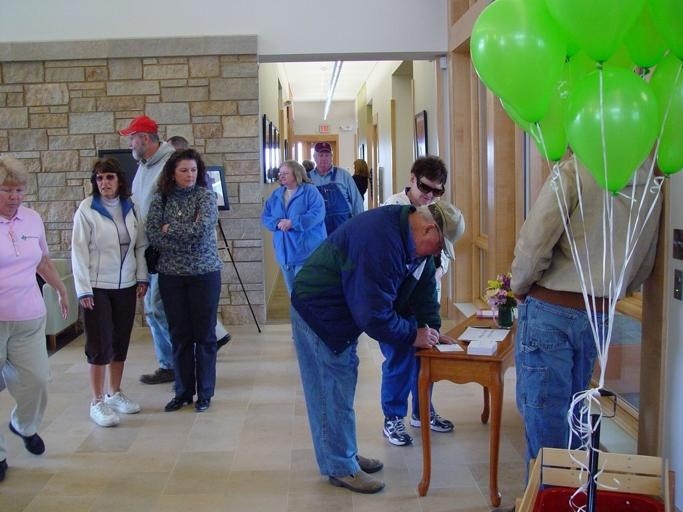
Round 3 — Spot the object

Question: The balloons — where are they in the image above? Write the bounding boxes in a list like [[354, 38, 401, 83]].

[[464, 1, 682, 196]]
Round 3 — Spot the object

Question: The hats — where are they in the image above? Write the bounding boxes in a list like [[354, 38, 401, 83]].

[[315, 142, 331, 153], [117, 116, 158, 136], [434, 200, 465, 262]]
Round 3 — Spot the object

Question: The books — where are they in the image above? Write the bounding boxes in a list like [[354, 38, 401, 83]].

[[475, 308, 519, 320]]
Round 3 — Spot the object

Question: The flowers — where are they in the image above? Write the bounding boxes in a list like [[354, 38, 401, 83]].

[[478, 270, 520, 310]]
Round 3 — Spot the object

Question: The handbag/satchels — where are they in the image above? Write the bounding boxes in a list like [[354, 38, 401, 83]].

[[145, 245, 160, 273]]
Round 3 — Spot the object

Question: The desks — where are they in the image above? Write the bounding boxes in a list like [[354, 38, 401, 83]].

[[412, 306, 519, 506]]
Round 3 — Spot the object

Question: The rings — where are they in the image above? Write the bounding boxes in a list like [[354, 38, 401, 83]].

[[84, 299, 88, 303]]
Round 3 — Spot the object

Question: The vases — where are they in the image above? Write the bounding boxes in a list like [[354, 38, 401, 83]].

[[492, 307, 514, 327]]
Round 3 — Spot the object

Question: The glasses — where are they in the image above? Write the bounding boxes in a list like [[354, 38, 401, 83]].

[[416, 177, 445, 197], [95, 175, 116, 181]]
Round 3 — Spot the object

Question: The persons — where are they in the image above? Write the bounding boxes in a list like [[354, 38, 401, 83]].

[[116, 115, 232, 413], [71, 154, 150, 428], [287, 199, 466, 495], [373, 155, 456, 449], [486, 150, 666, 512], [0, 155, 71, 486], [259, 138, 371, 341]]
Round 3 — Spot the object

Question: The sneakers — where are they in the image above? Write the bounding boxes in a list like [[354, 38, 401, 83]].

[[411, 411, 455, 432], [104, 392, 140, 414], [90, 400, 119, 426], [383, 416, 413, 446]]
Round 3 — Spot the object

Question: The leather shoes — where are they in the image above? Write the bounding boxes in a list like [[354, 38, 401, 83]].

[[329, 471, 385, 493], [357, 455, 383, 473], [139, 367, 176, 383], [215, 335, 231, 352], [164, 394, 211, 412], [8, 421, 45, 455], [0, 458, 8, 483]]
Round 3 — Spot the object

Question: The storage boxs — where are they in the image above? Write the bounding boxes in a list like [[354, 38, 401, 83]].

[[513, 445, 674, 512]]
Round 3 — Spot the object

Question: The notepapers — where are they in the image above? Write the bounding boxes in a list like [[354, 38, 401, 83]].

[[466, 340, 497, 355]]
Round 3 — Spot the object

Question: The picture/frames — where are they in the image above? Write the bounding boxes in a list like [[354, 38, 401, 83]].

[[204, 166, 230, 211], [263, 115, 290, 184], [413, 110, 428, 159]]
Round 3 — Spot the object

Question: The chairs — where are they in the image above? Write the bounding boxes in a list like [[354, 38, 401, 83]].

[[37, 257, 79, 352]]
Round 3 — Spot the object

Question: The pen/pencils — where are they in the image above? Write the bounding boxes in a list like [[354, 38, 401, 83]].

[[424, 323, 441, 346]]
